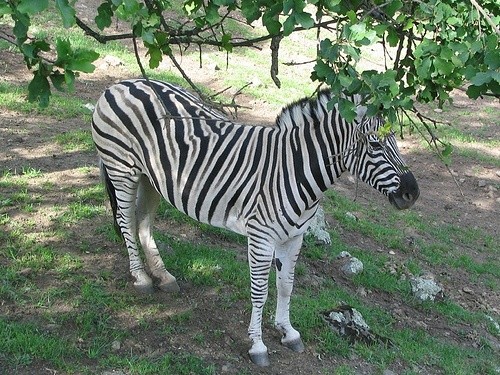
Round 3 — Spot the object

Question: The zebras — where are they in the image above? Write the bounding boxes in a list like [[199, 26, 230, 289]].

[[90, 77, 419, 367]]
